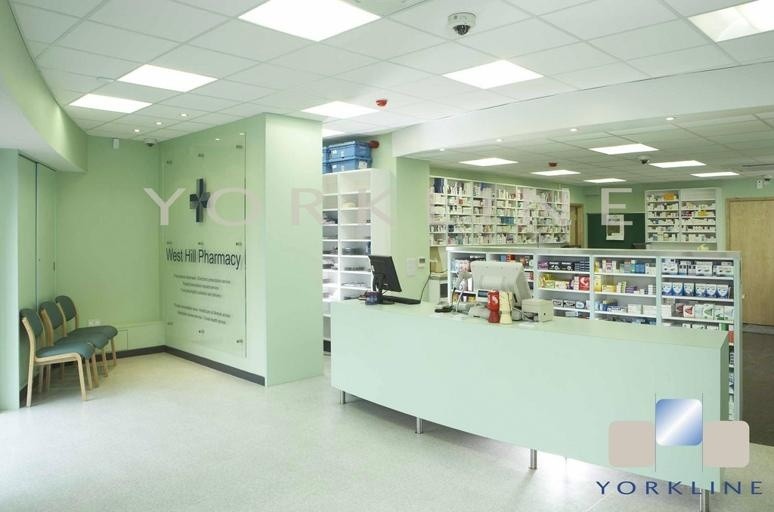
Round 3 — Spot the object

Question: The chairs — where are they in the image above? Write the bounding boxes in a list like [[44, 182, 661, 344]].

[[19, 296, 118, 407]]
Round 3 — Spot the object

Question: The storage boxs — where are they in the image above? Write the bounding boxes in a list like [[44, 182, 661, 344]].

[[321, 142, 373, 173]]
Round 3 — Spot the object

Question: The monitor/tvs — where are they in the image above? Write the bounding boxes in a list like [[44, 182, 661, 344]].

[[368, 254, 403, 304], [469, 261, 533, 307]]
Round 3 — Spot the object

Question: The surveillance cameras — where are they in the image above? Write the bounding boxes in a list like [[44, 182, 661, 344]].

[[450, 14, 474, 35], [639, 155, 650, 164]]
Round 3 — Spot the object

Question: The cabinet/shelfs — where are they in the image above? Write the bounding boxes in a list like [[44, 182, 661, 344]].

[[322, 167, 375, 342], [430, 177, 743, 421]]
